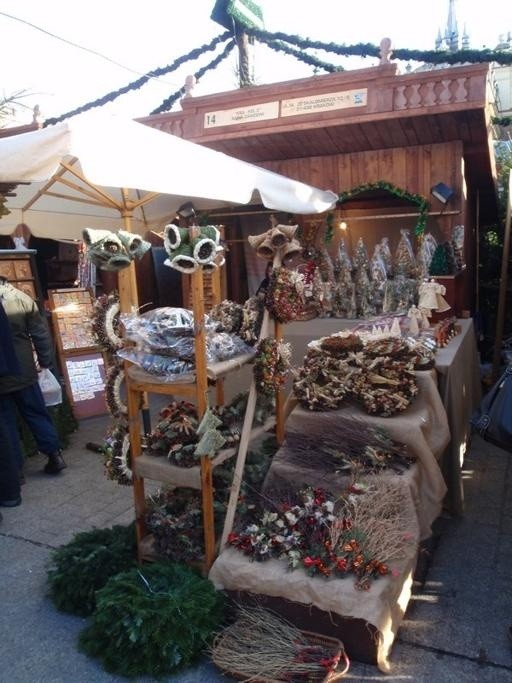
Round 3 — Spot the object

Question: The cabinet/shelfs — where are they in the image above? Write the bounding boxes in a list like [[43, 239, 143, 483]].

[[116, 226, 287, 580]]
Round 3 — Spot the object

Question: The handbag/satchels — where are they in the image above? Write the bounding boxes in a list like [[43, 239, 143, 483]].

[[469, 363, 512, 453]]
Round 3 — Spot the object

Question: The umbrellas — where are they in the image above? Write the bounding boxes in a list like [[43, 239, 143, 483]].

[[2, 103, 340, 450]]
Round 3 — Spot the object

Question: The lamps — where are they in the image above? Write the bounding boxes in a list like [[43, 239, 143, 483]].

[[430, 181, 455, 214]]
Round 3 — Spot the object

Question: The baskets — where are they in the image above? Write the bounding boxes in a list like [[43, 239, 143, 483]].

[[212, 624, 351, 682]]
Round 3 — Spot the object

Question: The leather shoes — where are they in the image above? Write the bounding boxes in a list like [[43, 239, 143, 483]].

[[0, 495, 22, 506], [44, 454, 67, 475]]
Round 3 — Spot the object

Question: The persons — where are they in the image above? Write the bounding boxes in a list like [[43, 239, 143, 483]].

[[0, 279, 69, 485], [0, 302, 23, 507]]
